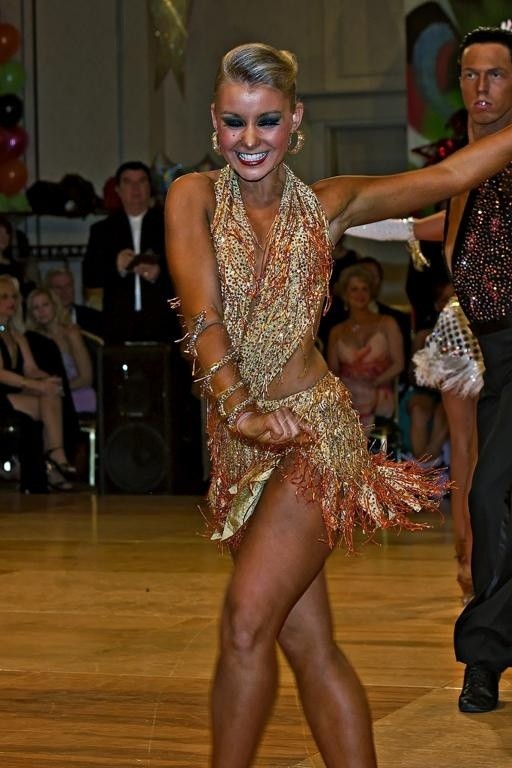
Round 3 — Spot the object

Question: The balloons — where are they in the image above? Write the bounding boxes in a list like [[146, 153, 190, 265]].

[[1, 18, 38, 213]]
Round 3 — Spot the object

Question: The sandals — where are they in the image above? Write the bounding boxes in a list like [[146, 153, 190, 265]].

[[49, 447, 77, 484], [45, 460, 74, 492]]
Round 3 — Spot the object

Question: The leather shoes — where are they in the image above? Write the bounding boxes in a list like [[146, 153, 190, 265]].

[[460, 661, 500, 714]]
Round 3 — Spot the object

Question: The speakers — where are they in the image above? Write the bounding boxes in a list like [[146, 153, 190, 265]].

[[95, 344, 200, 496]]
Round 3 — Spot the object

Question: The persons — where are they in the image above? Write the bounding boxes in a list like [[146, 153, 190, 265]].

[[2, 269, 104, 494], [313, 233, 450, 464], [84, 160, 205, 495], [165, 41, 512, 766], [440, 28, 512, 715], [338, 204, 492, 615]]
